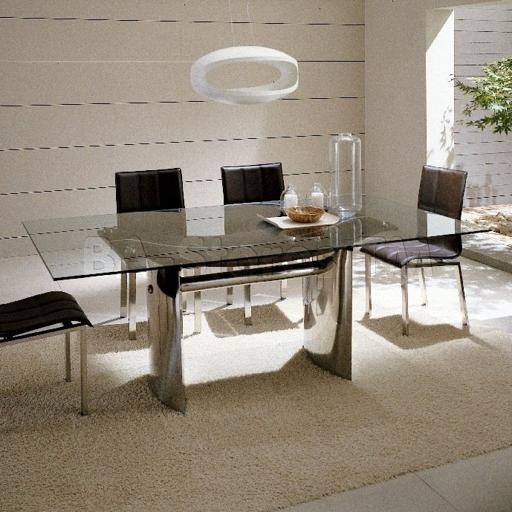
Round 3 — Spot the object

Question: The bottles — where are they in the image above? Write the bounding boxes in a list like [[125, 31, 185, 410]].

[[279, 181, 329, 216]]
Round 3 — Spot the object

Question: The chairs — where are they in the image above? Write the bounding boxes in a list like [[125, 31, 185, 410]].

[[106, 163, 289, 345], [359, 164, 470, 338]]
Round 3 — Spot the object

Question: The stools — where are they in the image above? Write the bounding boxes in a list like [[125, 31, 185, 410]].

[[0, 289, 93, 417]]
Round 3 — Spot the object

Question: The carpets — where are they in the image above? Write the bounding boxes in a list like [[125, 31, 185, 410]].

[[1, 285, 511, 511]]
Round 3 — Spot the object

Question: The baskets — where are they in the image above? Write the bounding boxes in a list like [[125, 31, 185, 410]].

[[286, 206, 323, 222]]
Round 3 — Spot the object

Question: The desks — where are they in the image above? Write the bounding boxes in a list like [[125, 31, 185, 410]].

[[19, 195, 485, 418]]
